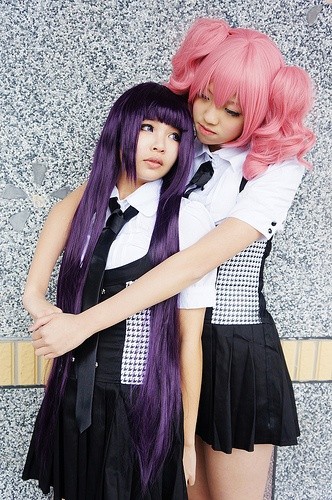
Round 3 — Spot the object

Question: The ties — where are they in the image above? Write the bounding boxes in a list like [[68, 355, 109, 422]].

[[180, 160, 217, 203], [72, 195, 144, 435]]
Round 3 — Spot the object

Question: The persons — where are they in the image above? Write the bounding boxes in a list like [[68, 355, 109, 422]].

[[20, 18, 316, 500], [19, 81, 216, 500]]
